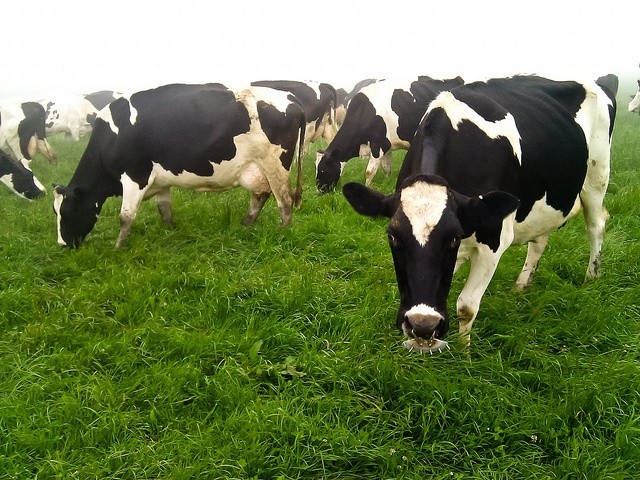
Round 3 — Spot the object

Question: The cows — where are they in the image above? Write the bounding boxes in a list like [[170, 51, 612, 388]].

[[38, 90, 123, 141], [251, 81, 337, 169], [335, 88, 349, 128], [315, 76, 465, 194], [0, 148, 47, 202], [52, 83, 305, 251], [0, 102, 58, 172], [345, 76, 434, 110], [342, 73, 619, 367]]
